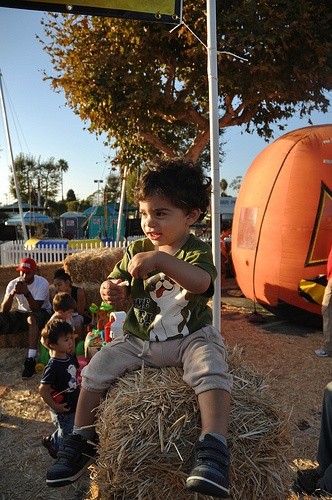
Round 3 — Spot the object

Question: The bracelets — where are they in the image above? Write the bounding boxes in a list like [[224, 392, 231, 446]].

[[24, 291, 32, 299]]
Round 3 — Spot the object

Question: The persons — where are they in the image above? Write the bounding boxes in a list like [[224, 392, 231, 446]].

[[293, 381, 332, 496], [220, 220, 232, 289], [45, 157, 234, 497], [314, 248, 332, 356], [0, 258, 88, 460]]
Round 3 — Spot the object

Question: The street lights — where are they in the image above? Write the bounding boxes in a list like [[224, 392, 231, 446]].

[[94, 180, 103, 206]]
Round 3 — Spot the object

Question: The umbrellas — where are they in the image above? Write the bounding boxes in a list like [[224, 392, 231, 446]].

[[0, 199, 139, 242]]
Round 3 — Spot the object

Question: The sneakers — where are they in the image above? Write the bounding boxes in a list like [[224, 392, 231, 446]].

[[21, 357, 36, 378], [314, 349, 329, 356], [43, 436, 59, 459], [46, 433, 98, 487], [185, 434, 230, 496]]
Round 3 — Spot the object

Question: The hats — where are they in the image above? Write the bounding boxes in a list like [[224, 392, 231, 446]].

[[16, 258, 36, 272]]
